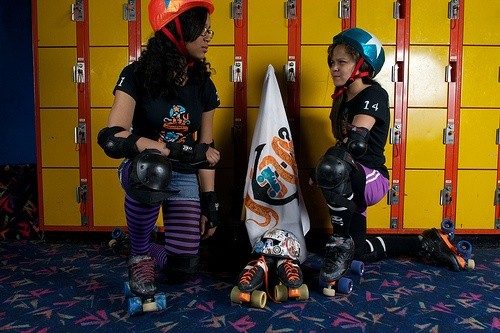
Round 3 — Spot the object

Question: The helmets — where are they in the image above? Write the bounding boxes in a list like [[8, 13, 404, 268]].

[[334, 28, 385, 78], [148, 0, 214, 32], [250, 229, 300, 258]]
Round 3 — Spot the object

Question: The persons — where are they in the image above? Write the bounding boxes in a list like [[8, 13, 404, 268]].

[[309, 28, 476, 296], [98, 0, 220, 313]]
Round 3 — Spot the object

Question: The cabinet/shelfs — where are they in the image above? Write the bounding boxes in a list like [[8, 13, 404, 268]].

[[34, 0, 500, 235]]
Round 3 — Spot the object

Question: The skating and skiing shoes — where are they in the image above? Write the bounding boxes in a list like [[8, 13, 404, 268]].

[[122, 247, 166, 315], [231, 257, 273, 308], [415, 219, 476, 272], [107, 223, 158, 251], [274, 258, 311, 302], [319, 234, 365, 296]]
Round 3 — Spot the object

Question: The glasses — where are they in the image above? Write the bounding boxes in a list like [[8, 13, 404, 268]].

[[201, 26, 214, 40]]
